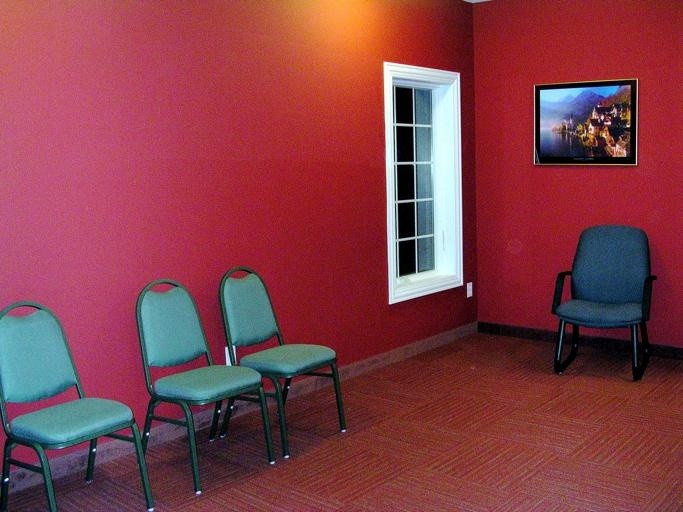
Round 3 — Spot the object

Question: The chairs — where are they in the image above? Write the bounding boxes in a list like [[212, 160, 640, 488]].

[[551, 223, 658, 382]]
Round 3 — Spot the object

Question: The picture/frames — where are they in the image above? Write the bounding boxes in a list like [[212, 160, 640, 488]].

[[533, 78, 640, 167]]
